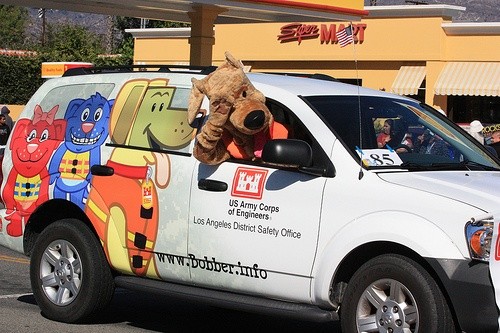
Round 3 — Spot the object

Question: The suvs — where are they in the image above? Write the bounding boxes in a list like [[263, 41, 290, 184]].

[[0, 63, 500, 333]]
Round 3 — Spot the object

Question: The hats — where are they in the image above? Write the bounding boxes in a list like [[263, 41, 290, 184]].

[[469, 120, 484, 133]]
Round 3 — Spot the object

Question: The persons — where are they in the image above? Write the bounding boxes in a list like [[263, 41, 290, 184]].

[[0, 106, 14, 154], [377, 119, 484, 160]]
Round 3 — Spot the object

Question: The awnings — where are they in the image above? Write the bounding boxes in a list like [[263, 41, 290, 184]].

[[434, 61, 500, 97], [391, 66, 426, 95]]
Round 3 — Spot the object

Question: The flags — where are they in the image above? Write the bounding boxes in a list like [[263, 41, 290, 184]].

[[336, 26, 353, 48]]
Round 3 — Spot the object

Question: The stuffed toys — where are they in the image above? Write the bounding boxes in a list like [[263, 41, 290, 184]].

[[187, 51, 288, 166]]
